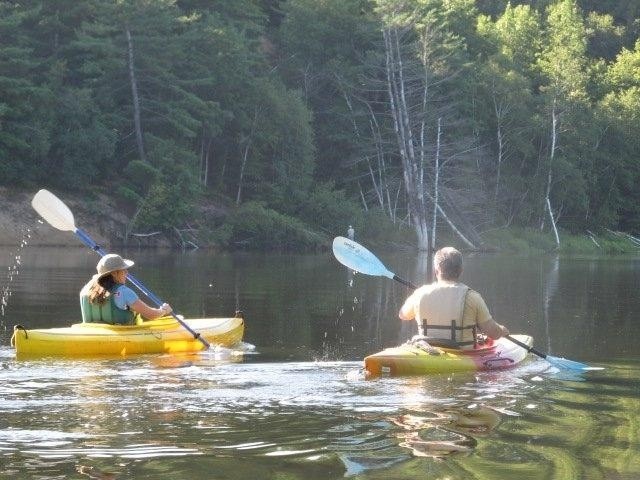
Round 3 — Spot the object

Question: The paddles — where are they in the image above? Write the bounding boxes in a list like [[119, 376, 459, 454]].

[[333, 236, 587, 370], [32, 188, 223, 353]]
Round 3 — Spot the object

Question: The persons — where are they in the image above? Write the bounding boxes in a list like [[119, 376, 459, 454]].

[[399, 247, 509, 351], [347, 226, 355, 241], [80, 254, 173, 326]]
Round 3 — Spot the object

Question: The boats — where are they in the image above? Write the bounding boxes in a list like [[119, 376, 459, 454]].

[[364, 334, 534, 375], [14, 318, 245, 362]]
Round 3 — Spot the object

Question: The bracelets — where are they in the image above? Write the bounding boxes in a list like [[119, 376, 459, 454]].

[[159, 305, 168, 315]]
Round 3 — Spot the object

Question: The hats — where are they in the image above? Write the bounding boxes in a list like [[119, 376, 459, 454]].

[[93, 254, 134, 282]]
